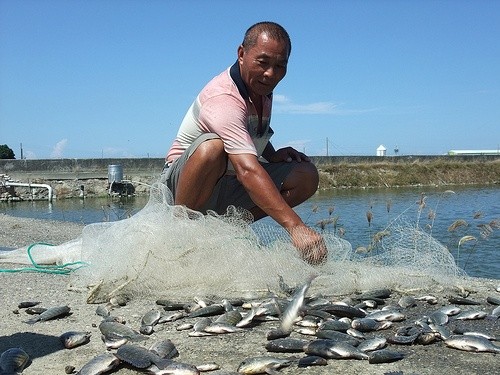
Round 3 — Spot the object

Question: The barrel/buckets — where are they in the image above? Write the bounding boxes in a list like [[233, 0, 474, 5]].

[[106, 164, 123, 195]]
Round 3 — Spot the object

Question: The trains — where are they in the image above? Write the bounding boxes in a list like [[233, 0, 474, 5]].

[[444, 149, 500, 154]]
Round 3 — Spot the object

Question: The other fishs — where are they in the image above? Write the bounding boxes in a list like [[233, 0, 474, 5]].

[[0, 273, 500, 375]]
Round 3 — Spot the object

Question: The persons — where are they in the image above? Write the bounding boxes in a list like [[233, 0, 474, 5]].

[[158, 22, 329, 268]]
